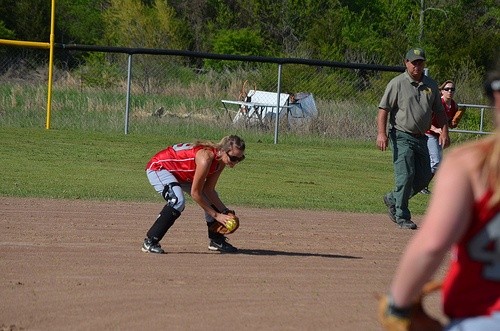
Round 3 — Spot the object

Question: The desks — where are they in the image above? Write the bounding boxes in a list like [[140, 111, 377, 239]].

[[221, 100, 295, 129]]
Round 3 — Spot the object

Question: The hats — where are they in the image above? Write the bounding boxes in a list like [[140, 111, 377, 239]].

[[405, 47, 425, 62]]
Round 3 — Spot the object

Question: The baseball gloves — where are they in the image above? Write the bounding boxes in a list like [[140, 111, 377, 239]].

[[209, 211, 240, 235]]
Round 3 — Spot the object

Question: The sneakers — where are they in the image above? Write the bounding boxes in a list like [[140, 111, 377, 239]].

[[384, 196, 396, 223], [141, 238, 166, 254], [207, 240, 238, 252], [420, 188, 431, 194], [398, 220, 417, 230]]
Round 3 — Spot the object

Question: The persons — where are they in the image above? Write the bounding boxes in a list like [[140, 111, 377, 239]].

[[376, 48, 441, 228], [141, 135, 246, 253], [379, 59, 500, 331], [421, 80, 460, 194]]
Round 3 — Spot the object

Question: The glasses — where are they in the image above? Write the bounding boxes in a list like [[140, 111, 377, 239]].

[[225, 151, 245, 162], [442, 87, 455, 91]]
